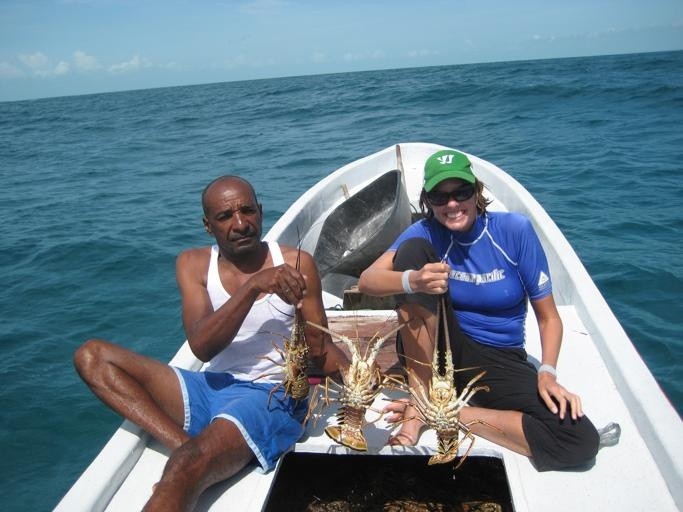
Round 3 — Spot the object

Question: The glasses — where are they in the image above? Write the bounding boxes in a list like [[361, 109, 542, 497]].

[[421, 184, 475, 208]]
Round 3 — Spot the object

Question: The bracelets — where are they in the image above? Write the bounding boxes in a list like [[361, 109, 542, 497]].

[[401, 269, 416, 295], [536, 364, 557, 378]]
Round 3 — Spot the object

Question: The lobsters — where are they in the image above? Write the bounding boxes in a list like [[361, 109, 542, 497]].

[[248, 224, 313, 423], [297, 277, 408, 454], [376, 231, 494, 481]]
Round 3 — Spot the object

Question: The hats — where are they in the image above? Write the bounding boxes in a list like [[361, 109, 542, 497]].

[[419, 148, 475, 194]]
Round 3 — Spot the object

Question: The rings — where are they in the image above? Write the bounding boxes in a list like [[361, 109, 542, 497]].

[[282, 288, 291, 295]]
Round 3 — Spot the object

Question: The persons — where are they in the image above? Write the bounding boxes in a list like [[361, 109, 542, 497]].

[[356, 148, 601, 474], [73, 175, 384, 512]]
